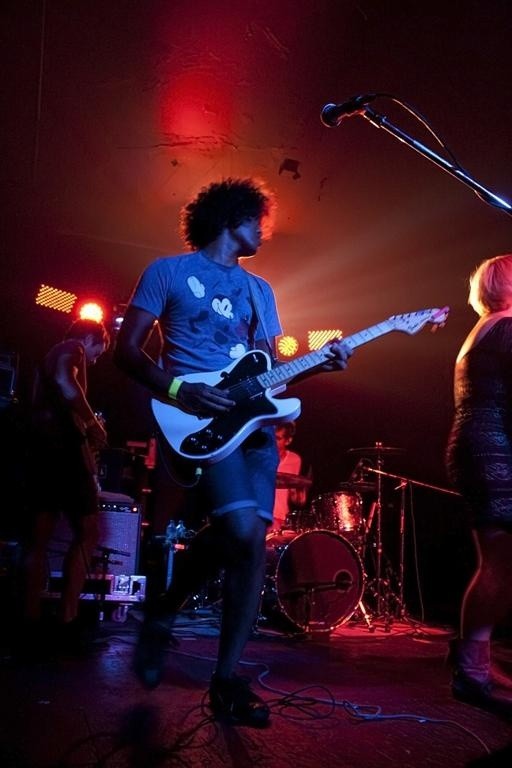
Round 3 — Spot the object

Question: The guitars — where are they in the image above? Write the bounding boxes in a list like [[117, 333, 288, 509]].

[[68, 407, 105, 477], [150, 306, 450, 463]]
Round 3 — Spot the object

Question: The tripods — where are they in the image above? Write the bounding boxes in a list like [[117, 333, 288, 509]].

[[363, 457, 412, 624]]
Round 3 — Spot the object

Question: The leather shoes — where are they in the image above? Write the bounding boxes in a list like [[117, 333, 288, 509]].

[[210, 672, 270, 724]]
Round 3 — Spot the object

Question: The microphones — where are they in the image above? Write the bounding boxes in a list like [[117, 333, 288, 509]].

[[348, 459, 364, 484], [320, 93, 374, 126]]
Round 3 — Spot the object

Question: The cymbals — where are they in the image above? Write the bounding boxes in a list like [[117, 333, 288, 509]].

[[341, 479, 378, 493], [272, 470, 312, 488], [342, 446, 406, 459]]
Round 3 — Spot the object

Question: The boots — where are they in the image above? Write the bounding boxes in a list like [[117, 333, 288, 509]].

[[449, 639, 511, 716]]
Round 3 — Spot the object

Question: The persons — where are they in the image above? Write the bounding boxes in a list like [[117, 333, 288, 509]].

[[112, 173, 357, 725], [268, 421, 313, 533], [441, 253, 510, 708], [327, 461, 393, 598], [16, 320, 115, 653]]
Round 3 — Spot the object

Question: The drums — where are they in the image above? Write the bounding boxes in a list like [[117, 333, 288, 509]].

[[309, 490, 366, 541], [261, 529, 367, 637]]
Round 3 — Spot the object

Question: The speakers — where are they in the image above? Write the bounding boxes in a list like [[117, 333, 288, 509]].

[[46, 492, 140, 594]]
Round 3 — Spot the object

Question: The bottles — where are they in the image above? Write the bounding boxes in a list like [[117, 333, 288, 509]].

[[165, 517, 185, 542]]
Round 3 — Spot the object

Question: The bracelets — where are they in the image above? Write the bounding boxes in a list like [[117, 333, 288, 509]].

[[168, 377, 184, 402], [86, 418, 98, 426]]
